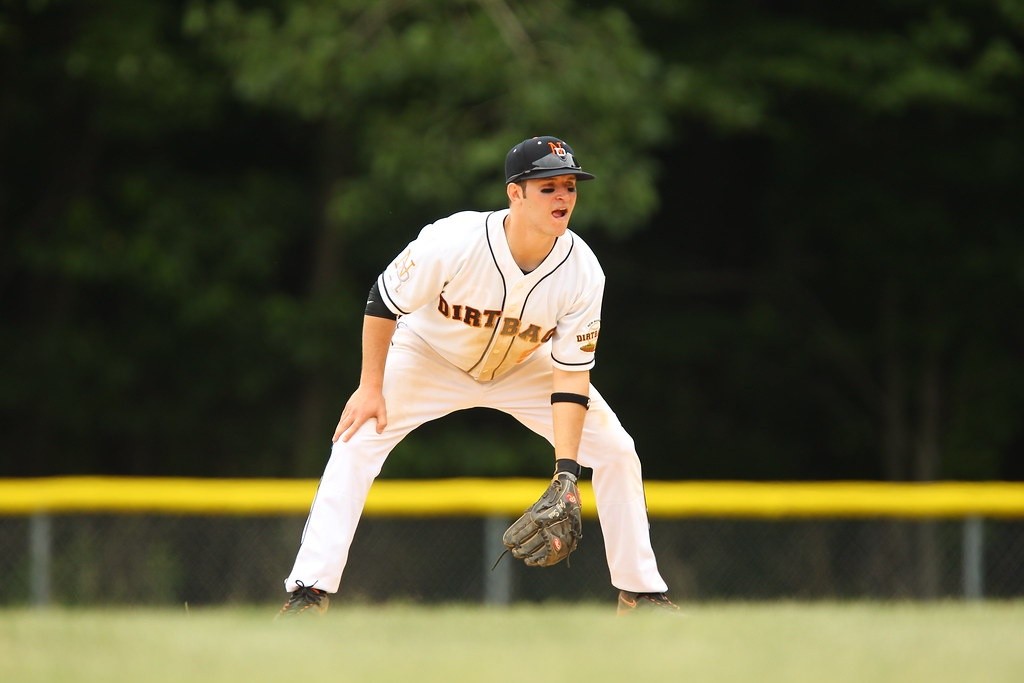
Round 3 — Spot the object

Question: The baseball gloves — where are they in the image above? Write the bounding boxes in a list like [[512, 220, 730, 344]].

[[488, 470, 585, 570]]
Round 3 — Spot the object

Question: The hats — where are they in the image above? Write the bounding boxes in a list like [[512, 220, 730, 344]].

[[505, 136, 595, 183]]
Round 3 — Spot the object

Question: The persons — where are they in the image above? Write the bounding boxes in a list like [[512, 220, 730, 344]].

[[270, 136, 680, 618]]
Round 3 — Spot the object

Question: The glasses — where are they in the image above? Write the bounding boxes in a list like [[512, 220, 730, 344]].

[[507, 151, 582, 182]]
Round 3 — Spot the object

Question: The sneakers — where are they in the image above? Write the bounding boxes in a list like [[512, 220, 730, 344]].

[[274, 579, 329, 625], [616, 591, 685, 619]]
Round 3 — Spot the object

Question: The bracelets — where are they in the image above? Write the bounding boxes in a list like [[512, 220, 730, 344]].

[[553, 458, 581, 476], [551, 392, 590, 408]]
